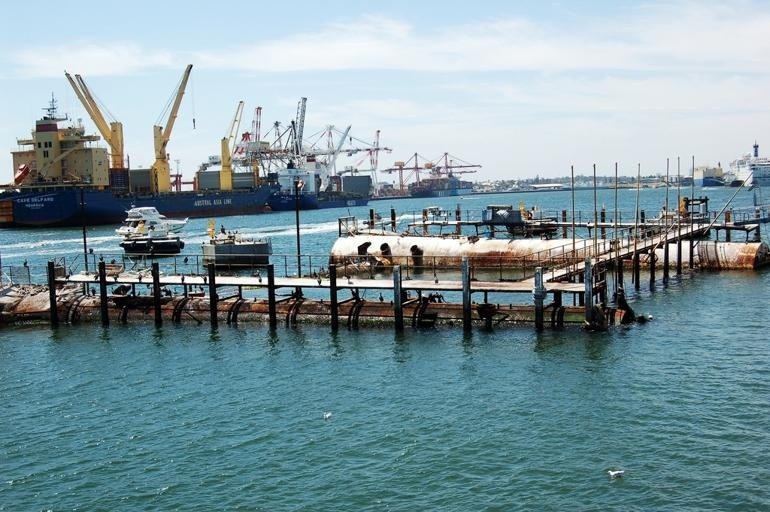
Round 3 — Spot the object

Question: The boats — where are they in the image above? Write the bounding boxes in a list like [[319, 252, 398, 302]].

[[506, 217, 559, 235], [116, 230, 185, 258], [197, 234, 273, 269], [116, 204, 189, 238]]
